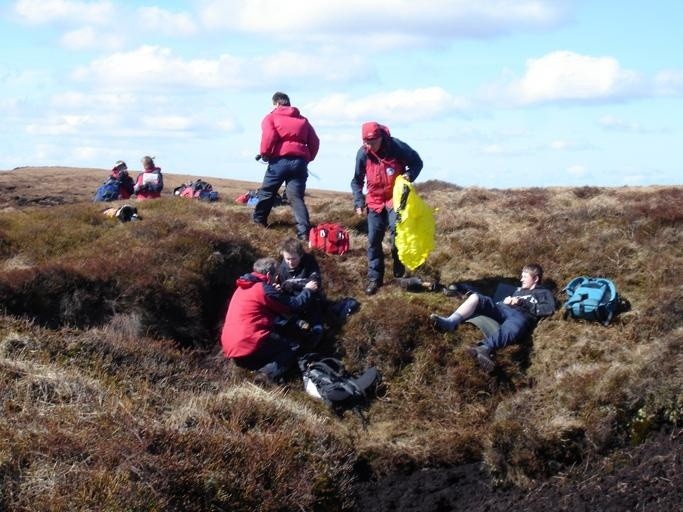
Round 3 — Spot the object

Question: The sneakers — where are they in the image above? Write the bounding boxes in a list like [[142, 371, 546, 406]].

[[401, 277, 420, 290], [365, 282, 382, 295], [296, 234, 308, 241], [250, 373, 276, 390], [394, 272, 407, 284], [469, 345, 495, 373]]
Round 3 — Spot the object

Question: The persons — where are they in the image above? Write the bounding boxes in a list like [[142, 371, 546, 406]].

[[251, 92, 319, 241], [352, 122, 423, 295], [133, 157, 162, 200], [430, 263, 555, 372], [275, 238, 322, 299], [221, 257, 318, 391], [104, 160, 133, 199]]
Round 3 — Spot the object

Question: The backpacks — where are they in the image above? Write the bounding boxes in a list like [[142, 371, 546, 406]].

[[560, 276, 618, 326], [298, 350, 379, 407], [95, 175, 123, 202], [173, 179, 217, 202], [308, 223, 349, 257], [235, 188, 292, 207]]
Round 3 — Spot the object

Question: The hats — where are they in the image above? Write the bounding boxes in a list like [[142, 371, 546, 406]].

[[363, 123, 380, 140], [112, 160, 127, 169], [139, 157, 155, 163]]
